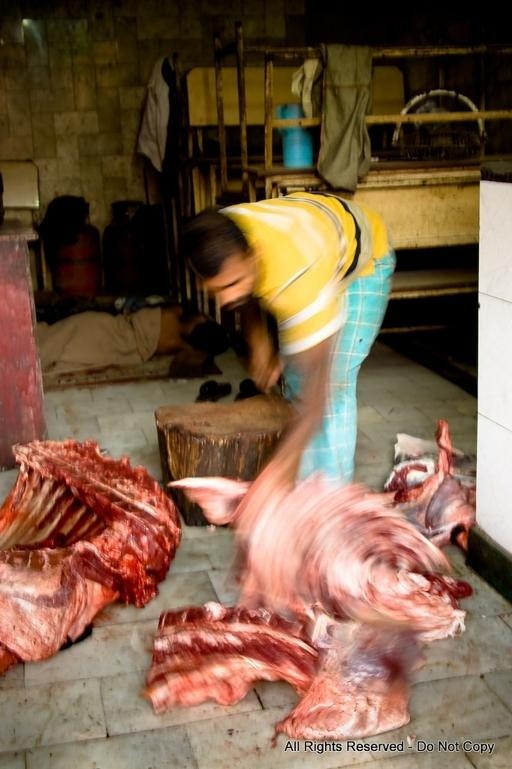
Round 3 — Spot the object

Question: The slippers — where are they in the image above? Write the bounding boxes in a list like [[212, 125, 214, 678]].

[[194, 380, 231, 404], [236, 378, 261, 398]]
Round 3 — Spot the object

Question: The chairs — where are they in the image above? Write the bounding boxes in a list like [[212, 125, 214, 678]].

[[1, 158, 45, 294]]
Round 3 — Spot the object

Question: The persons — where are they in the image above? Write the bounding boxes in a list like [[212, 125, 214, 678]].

[[179, 190, 399, 606]]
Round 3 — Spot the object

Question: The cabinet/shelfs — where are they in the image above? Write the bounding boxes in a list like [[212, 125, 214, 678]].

[[162, 28, 512, 338]]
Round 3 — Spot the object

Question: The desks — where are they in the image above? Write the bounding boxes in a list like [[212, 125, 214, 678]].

[[0, 218, 48, 471]]
